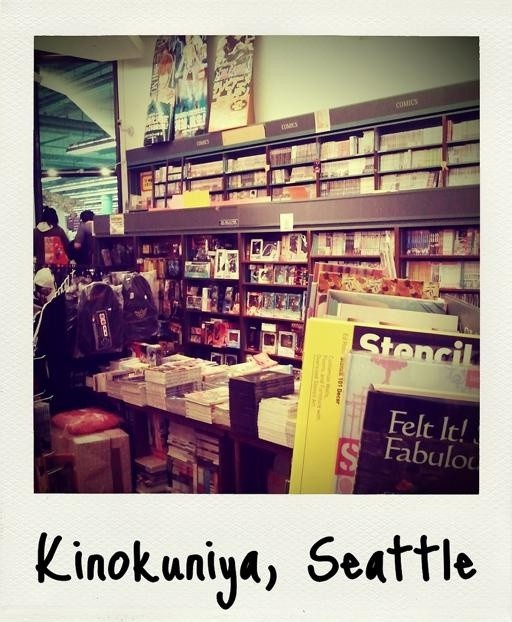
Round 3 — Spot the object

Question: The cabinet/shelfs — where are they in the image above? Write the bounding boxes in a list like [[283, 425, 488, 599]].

[[130, 405, 293, 494]]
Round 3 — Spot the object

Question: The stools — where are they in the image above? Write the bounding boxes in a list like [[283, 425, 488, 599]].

[[49, 427, 130, 493]]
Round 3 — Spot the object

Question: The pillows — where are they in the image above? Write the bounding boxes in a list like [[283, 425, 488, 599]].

[[52, 406, 123, 436]]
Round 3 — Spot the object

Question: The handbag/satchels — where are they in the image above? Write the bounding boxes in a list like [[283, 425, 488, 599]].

[[76, 281, 125, 358], [121, 274, 162, 343], [44, 236, 69, 266]]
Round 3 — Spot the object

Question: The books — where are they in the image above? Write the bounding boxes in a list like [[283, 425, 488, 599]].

[[142, 37, 255, 148], [80, 114, 479, 493]]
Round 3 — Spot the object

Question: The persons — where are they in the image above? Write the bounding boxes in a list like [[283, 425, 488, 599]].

[[68, 210, 96, 260], [34, 207, 76, 274]]
